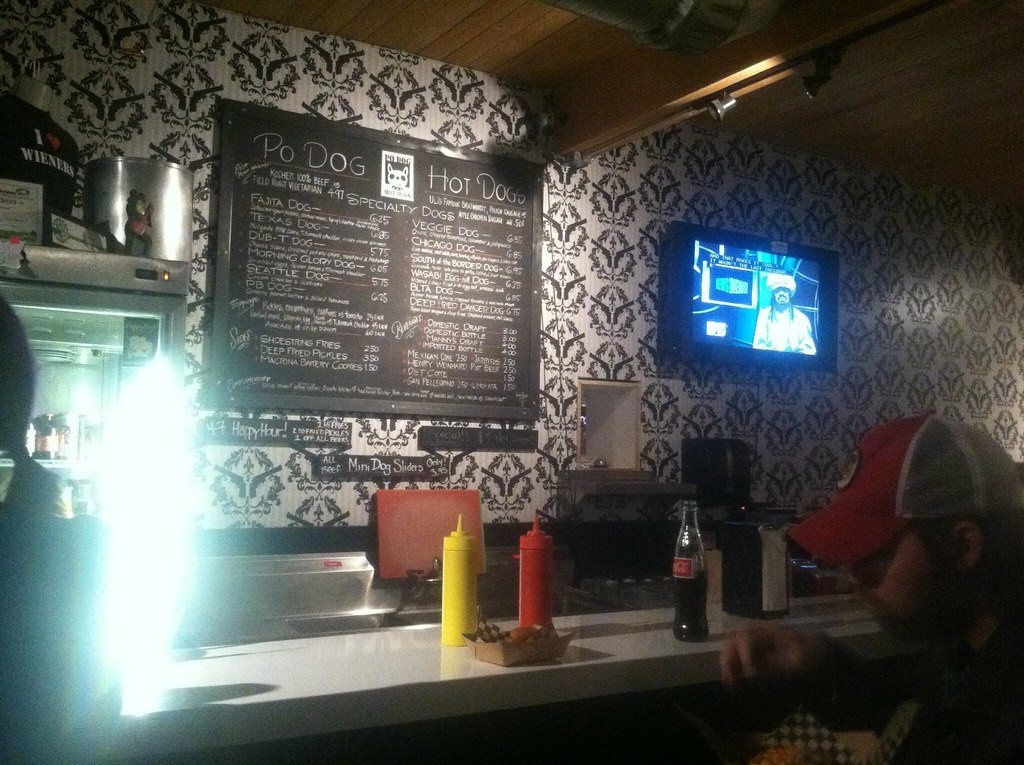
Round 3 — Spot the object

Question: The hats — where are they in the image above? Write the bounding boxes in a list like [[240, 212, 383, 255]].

[[787, 412, 1024, 566], [768, 274, 795, 300]]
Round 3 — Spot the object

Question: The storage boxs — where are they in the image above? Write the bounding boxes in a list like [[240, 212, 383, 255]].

[[461, 622, 574, 667]]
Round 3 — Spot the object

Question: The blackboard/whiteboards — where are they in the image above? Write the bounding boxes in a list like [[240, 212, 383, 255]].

[[316, 453, 450, 481], [200, 418, 353, 447], [204, 98, 544, 421], [418, 425, 539, 453]]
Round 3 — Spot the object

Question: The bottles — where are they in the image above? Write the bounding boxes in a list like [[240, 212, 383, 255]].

[[519, 513, 553, 629], [40, 412, 56, 459], [671, 501, 709, 641], [442, 514, 478, 648]]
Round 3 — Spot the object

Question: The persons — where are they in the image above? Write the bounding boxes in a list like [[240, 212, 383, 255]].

[[124, 189, 154, 257], [720, 414, 1024, 765], [753, 273, 817, 355], [0, 297, 121, 765]]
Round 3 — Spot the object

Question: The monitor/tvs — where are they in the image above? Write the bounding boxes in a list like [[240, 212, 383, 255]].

[[658, 222, 839, 374]]
